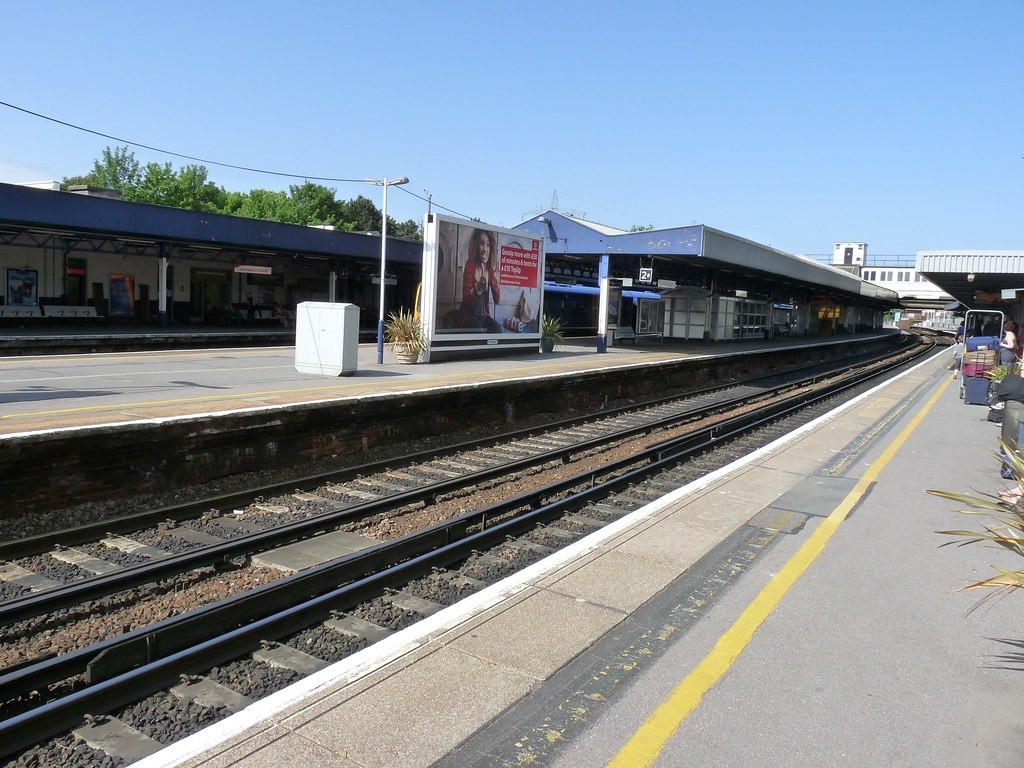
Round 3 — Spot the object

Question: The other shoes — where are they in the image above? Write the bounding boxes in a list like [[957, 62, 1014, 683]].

[[953, 375, 957, 379]]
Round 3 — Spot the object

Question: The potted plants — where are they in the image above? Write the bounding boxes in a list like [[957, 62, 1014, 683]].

[[380, 306, 431, 365], [539, 312, 567, 353]]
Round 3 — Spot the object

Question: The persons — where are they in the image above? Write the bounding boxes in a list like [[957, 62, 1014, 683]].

[[952, 315, 1024, 379], [459, 228, 502, 334]]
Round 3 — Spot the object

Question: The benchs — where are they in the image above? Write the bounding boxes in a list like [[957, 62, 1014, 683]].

[[614, 327, 637, 346]]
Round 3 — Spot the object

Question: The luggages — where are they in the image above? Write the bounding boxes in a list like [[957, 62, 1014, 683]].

[[963, 334, 1000, 386], [963, 353, 990, 405]]
